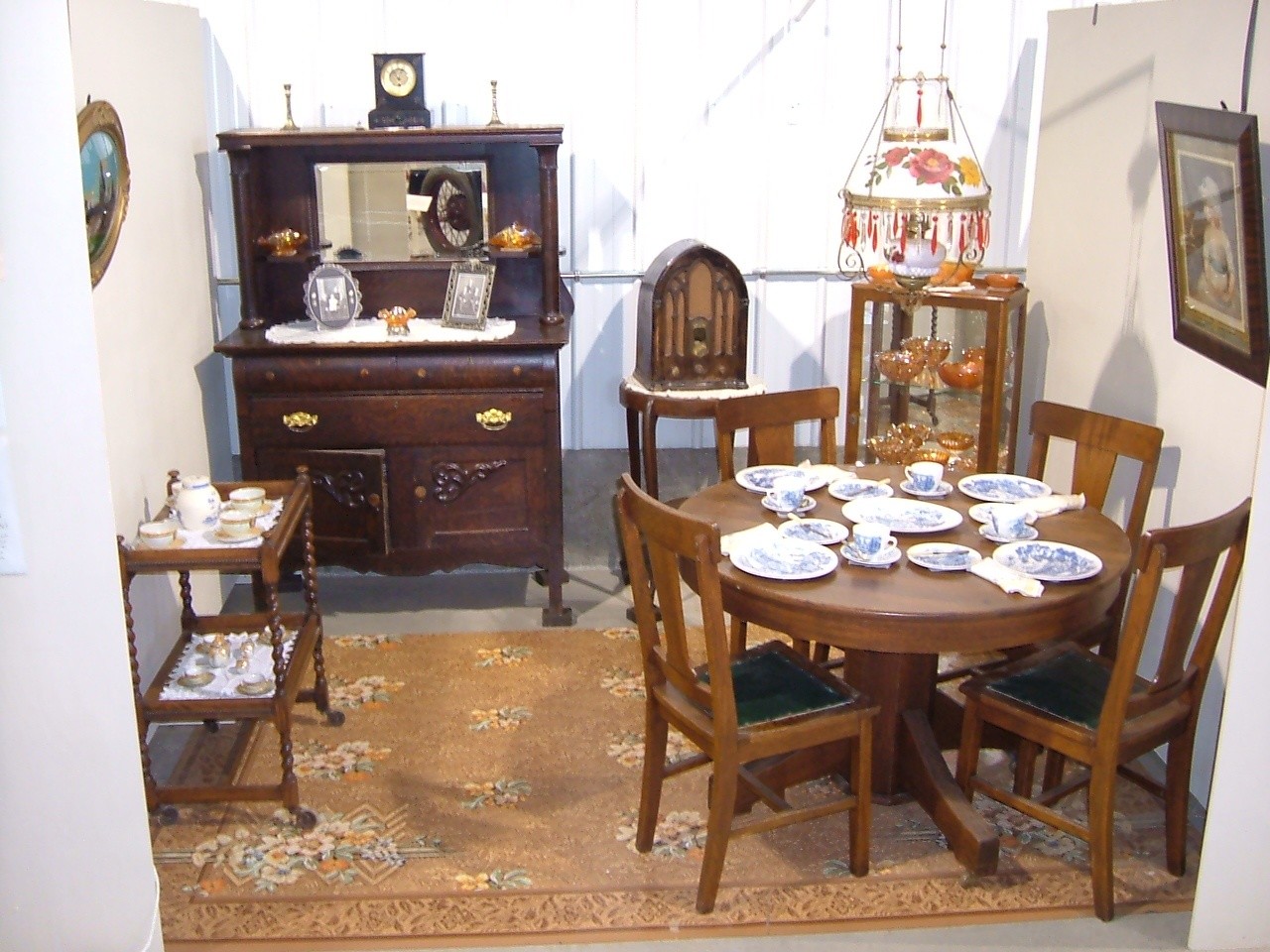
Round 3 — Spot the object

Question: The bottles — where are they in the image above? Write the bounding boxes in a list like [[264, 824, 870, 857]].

[[176, 475, 222, 534]]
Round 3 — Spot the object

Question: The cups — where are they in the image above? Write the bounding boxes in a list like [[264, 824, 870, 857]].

[[219, 510, 258, 536], [175, 623, 294, 695], [766, 476, 806, 507], [904, 461, 944, 492], [140, 520, 177, 550], [852, 522, 898, 560], [229, 487, 266, 511]]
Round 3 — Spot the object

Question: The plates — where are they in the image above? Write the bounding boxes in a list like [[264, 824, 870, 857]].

[[957, 474, 1053, 504], [979, 524, 1039, 544], [840, 542, 901, 569], [899, 479, 954, 498], [136, 502, 272, 550], [259, 630, 291, 645], [968, 502, 1039, 526], [213, 530, 259, 543], [196, 639, 231, 652], [734, 464, 827, 493], [827, 478, 894, 501], [761, 494, 816, 518], [177, 672, 214, 688], [841, 498, 964, 534], [992, 540, 1103, 582], [729, 539, 839, 580], [778, 519, 850, 545], [238, 679, 272, 695], [906, 543, 982, 570]]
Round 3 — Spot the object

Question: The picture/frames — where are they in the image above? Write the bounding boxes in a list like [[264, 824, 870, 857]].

[[77, 100, 131, 290], [440, 256, 497, 332], [303, 262, 363, 334], [1155, 101, 1270, 389]]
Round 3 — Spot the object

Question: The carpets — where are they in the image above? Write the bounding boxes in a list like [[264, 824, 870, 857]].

[[139, 620, 1204, 952]]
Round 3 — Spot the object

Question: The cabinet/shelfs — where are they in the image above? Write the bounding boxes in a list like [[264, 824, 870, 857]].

[[843, 273, 1028, 475], [213, 127, 575, 627]]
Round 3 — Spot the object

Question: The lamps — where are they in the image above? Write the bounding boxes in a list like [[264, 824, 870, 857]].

[[834, 0, 993, 317]]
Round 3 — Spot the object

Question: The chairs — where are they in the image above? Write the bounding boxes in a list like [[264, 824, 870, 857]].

[[613, 471, 886, 916], [955, 496, 1258, 922], [933, 398, 1164, 802], [714, 383, 849, 682]]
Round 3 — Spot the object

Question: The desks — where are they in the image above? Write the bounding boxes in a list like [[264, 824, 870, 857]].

[[676, 464, 1135, 879], [618, 377, 764, 627]]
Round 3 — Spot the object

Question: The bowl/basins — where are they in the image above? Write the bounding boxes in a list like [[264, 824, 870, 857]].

[[865, 262, 1019, 466]]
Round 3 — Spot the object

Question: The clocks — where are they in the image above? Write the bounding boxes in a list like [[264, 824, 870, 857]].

[[367, 53, 430, 130]]
